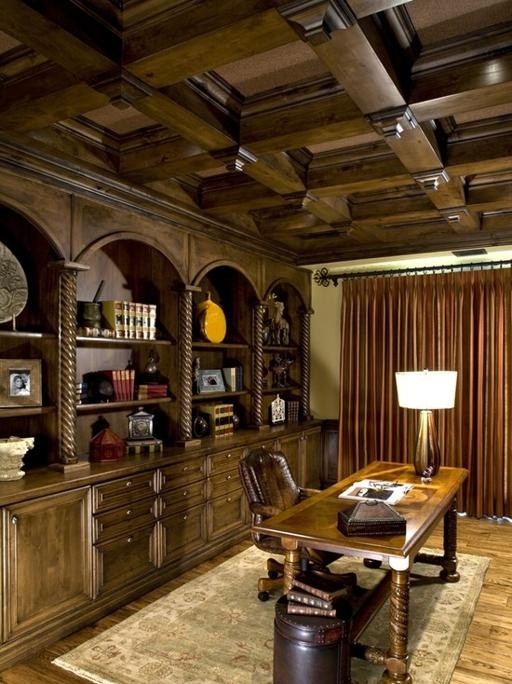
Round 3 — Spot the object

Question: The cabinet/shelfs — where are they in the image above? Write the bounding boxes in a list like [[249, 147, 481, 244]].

[[0, 134, 326, 672]]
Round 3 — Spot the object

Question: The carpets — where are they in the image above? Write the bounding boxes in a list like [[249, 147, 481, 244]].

[[50, 545, 493, 684]]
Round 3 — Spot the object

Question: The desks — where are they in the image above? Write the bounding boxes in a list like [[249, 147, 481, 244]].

[[253, 459, 473, 683]]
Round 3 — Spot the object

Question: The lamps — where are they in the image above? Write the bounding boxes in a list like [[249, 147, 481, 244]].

[[393, 366, 459, 474]]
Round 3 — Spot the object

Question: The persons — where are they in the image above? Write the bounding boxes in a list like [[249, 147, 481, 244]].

[[11, 373, 30, 395], [132, 418, 149, 437]]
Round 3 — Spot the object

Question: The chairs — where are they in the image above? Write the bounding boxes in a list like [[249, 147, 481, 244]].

[[238, 447, 357, 602]]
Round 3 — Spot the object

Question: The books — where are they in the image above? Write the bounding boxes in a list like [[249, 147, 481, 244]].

[[285, 570, 349, 618]]
